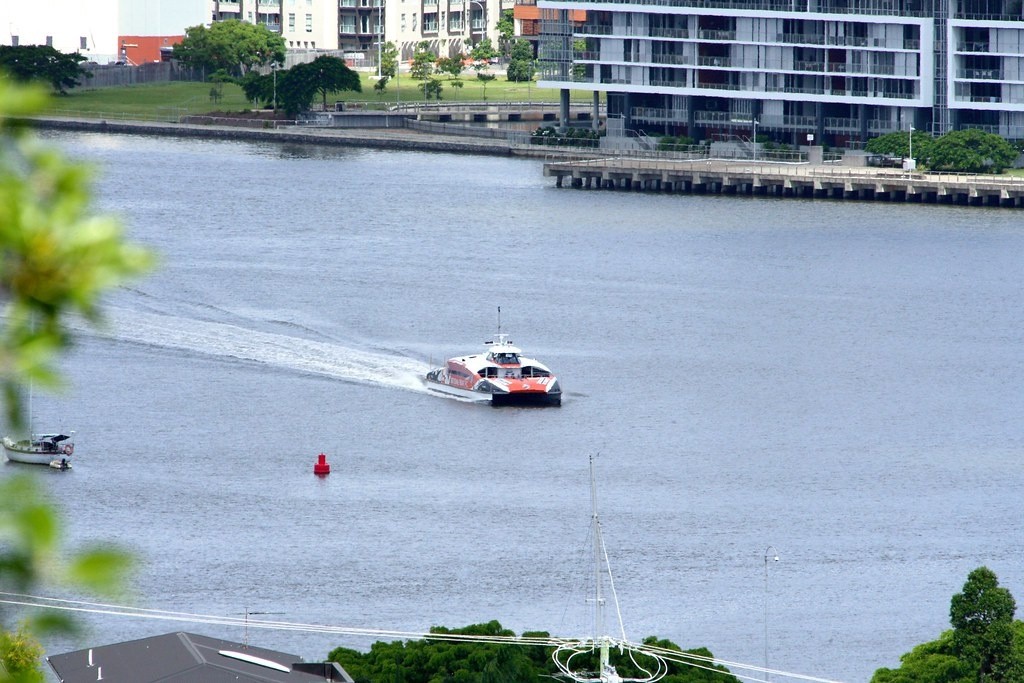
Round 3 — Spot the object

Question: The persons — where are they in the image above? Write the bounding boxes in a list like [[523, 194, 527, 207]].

[[492, 352, 520, 364]]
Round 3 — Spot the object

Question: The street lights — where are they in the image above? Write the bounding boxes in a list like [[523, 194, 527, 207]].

[[908, 123, 916, 158], [753, 117, 760, 161], [471, 0, 484, 43]]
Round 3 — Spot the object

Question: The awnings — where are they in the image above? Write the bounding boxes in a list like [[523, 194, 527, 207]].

[[32, 434, 71, 444]]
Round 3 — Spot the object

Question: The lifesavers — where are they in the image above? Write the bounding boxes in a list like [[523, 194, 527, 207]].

[[64, 445, 73, 455]]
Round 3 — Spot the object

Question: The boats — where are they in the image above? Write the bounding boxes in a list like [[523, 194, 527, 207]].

[[50, 459, 73, 471], [425, 305, 564, 408]]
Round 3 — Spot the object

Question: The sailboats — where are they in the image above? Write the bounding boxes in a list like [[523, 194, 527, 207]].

[[0, 300, 77, 465]]
